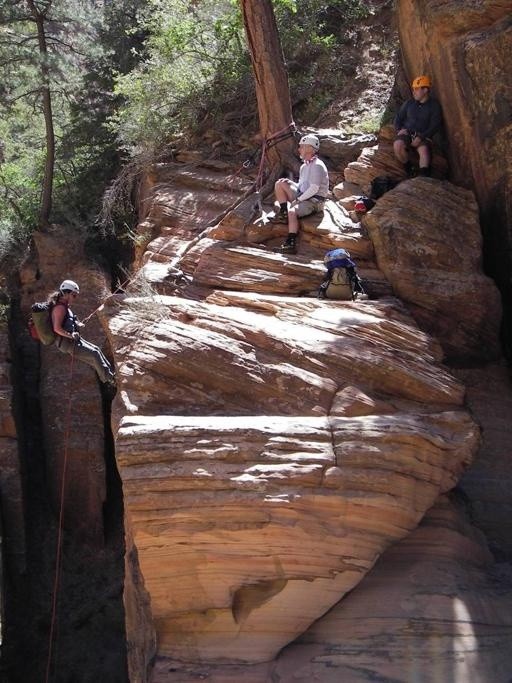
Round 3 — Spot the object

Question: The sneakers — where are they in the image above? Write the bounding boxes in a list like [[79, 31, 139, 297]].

[[267, 212, 288, 224], [272, 239, 297, 254], [406, 158, 430, 177]]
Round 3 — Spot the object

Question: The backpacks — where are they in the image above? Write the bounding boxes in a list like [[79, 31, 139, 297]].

[[318, 247, 369, 302], [30, 302, 69, 346]]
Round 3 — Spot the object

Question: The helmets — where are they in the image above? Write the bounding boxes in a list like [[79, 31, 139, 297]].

[[411, 75, 432, 88], [60, 279, 80, 294], [299, 133, 320, 149]]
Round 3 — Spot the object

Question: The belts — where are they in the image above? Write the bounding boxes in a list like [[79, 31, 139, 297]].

[[297, 188, 325, 201]]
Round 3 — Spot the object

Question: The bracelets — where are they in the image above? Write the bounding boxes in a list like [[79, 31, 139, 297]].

[[296, 197, 299, 204]]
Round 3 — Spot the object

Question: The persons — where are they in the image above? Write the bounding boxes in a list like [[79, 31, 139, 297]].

[[393, 75, 443, 179], [48, 279, 117, 388], [270, 134, 330, 255]]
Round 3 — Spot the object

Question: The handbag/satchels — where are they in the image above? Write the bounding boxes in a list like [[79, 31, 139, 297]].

[[367, 175, 396, 196]]
